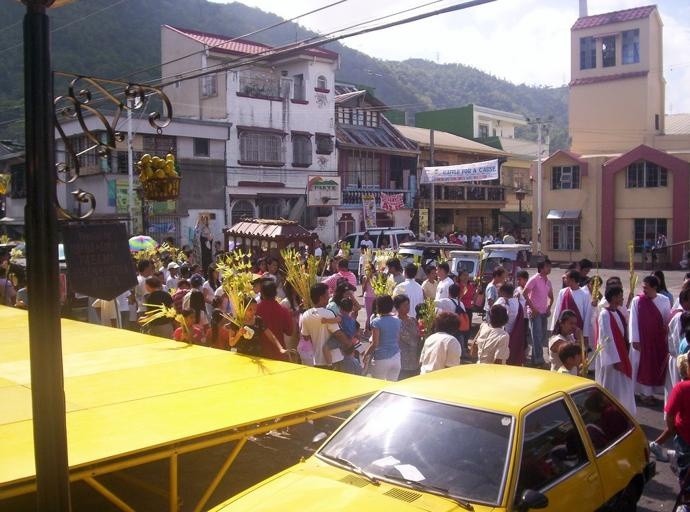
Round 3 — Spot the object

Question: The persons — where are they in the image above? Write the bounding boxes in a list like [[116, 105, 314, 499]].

[[92, 238, 293, 361], [643, 237, 654, 260], [467, 255, 602, 376], [298, 232, 473, 382], [593, 270, 690, 511], [1, 264, 29, 310], [424, 229, 528, 250], [651, 231, 668, 261]]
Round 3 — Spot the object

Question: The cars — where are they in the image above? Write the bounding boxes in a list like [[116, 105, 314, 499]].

[[204, 362, 657, 512]]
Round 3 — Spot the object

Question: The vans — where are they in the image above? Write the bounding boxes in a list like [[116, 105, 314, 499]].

[[327, 227, 418, 284]]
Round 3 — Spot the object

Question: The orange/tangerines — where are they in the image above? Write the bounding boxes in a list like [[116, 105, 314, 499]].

[[138, 154, 178, 197]]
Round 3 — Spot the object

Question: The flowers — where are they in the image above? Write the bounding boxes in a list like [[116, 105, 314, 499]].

[[417, 296, 439, 339], [624, 237, 640, 309], [471, 270, 486, 293], [214, 247, 254, 337], [577, 330, 610, 380], [361, 245, 449, 296], [131, 240, 190, 339], [588, 238, 604, 307], [279, 241, 350, 309]]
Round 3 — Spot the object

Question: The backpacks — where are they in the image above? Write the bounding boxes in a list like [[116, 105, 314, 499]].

[[451, 298, 469, 331]]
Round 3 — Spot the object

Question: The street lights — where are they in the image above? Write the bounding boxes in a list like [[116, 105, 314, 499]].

[[125, 87, 142, 239], [537, 127, 551, 258], [515, 187, 527, 242]]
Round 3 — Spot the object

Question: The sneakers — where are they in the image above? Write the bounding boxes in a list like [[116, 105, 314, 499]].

[[649, 441, 668, 461]]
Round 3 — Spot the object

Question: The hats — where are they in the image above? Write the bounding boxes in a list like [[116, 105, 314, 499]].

[[191, 275, 202, 286], [168, 262, 180, 270]]
